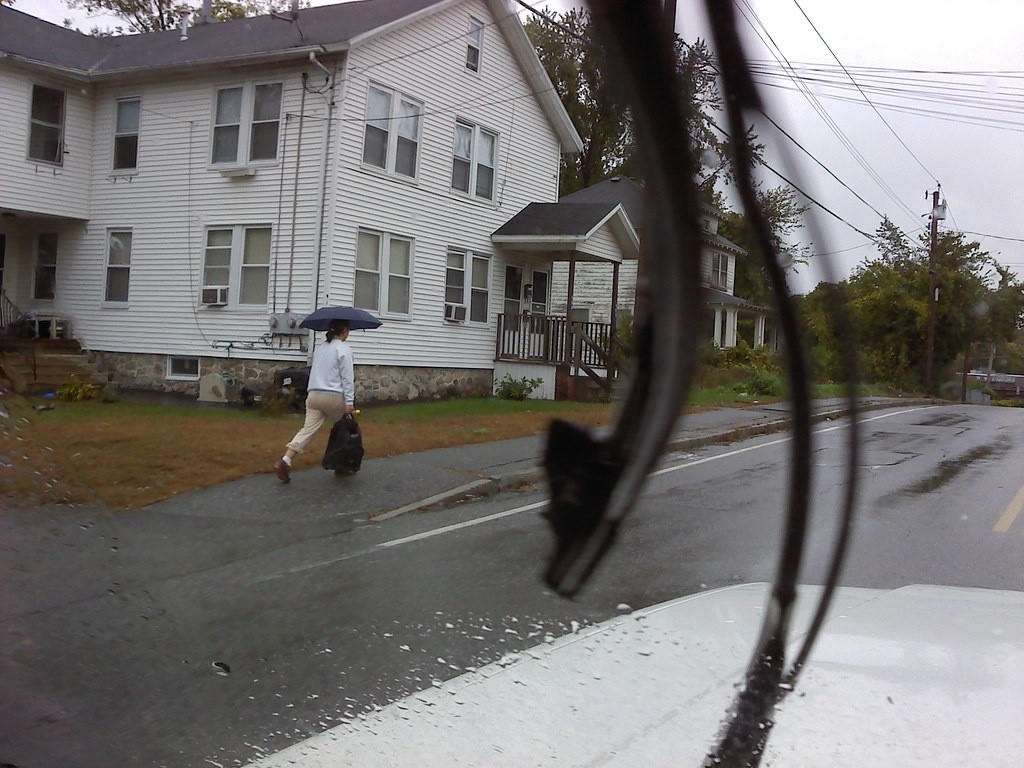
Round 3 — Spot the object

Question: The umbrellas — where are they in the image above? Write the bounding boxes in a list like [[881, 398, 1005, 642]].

[[298, 306, 382, 331]]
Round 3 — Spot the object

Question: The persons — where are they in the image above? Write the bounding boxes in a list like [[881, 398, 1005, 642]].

[[274, 320, 354, 482]]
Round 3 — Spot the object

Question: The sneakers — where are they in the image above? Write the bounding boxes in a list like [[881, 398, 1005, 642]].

[[274, 459, 291, 484]]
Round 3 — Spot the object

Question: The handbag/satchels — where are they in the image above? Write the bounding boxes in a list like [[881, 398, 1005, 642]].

[[322, 412, 364, 472]]
